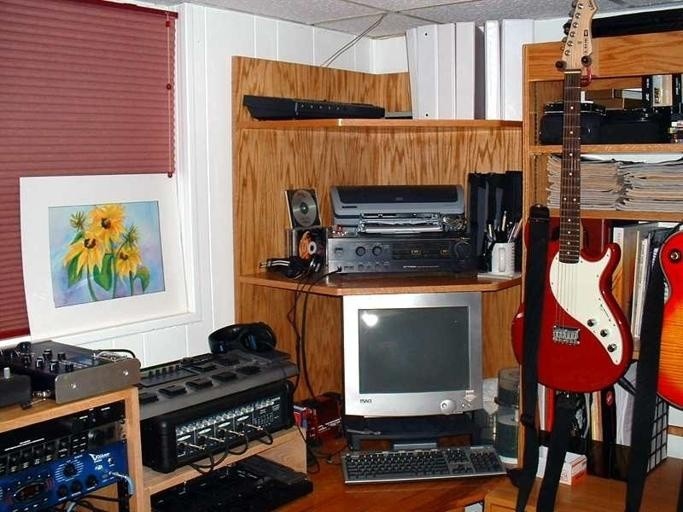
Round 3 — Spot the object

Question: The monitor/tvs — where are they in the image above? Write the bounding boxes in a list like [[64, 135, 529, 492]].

[[342, 291, 484, 440]]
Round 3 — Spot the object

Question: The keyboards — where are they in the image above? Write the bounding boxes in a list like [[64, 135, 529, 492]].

[[243, 95, 385, 119], [340, 445, 507, 484]]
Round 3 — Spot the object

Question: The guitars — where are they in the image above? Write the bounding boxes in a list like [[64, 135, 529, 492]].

[[656, 230, 683, 409], [511, 0, 634, 391]]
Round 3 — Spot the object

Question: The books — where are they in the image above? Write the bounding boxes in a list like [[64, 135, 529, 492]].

[[545, 155, 683, 213], [589, 222, 680, 446]]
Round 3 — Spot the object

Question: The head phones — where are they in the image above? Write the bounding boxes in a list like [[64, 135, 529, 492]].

[[259, 254, 321, 280], [208, 321, 277, 355]]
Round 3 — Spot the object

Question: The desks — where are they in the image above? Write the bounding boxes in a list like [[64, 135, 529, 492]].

[[486, 456, 683, 512], [1, 384, 508, 512]]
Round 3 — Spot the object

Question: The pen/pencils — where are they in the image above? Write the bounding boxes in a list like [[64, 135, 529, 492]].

[[488, 211, 523, 243]]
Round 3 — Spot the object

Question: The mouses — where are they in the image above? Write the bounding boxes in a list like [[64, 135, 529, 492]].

[[508, 468, 522, 488]]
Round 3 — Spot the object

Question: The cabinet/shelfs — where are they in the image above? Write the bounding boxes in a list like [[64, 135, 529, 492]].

[[230, 55, 524, 427], [517, 30, 683, 469]]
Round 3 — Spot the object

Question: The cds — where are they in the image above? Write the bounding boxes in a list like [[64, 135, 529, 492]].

[[291, 190, 317, 227]]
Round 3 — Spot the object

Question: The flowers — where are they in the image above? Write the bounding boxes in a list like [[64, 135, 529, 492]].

[[62, 204, 151, 301]]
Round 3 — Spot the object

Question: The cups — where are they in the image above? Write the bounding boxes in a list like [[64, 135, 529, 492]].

[[490, 242, 516, 276]]
